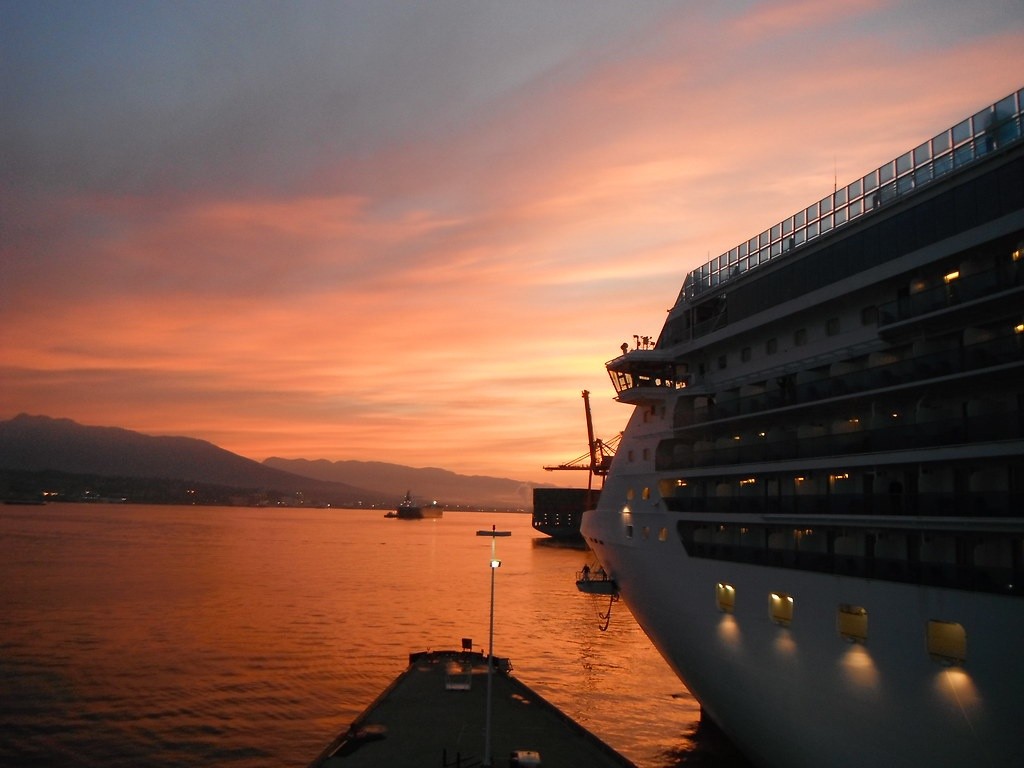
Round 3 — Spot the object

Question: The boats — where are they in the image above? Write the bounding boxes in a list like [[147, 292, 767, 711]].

[[384, 489, 444, 519], [576, 561, 619, 594], [311, 536, 635, 767], [476, 529, 511, 537], [532, 390, 626, 539]]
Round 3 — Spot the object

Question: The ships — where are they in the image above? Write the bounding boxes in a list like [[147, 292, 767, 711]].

[[581, 89, 1024, 768]]
